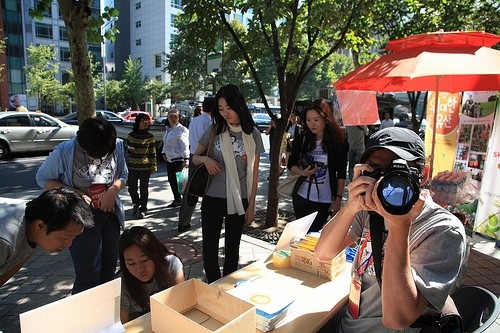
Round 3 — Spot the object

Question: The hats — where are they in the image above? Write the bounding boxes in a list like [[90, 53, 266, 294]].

[[167, 109, 179, 118], [360, 128, 427, 165]]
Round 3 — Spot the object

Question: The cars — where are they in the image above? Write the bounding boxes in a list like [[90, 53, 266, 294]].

[[119, 110, 154, 125], [155, 115, 169, 124], [0, 106, 82, 159], [57, 109, 130, 125], [253, 113, 273, 132]]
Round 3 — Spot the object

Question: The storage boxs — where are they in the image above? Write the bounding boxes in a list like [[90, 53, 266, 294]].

[[289, 246, 346, 281], [149, 278, 255, 333]]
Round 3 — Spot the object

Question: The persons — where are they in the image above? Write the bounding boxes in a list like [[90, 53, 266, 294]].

[[178, 97, 214, 231], [315, 127, 466, 333], [163, 109, 190, 207], [380, 112, 407, 129], [465, 95, 481, 118], [119, 226, 185, 323], [266, 110, 300, 179], [460, 123, 491, 181], [0, 188, 95, 288], [193, 84, 265, 284], [125, 113, 157, 218], [347, 125, 373, 179], [287, 99, 347, 233], [36, 117, 128, 295]]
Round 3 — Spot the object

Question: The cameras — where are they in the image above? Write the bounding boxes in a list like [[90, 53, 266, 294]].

[[363, 159, 424, 216], [299, 153, 325, 170]]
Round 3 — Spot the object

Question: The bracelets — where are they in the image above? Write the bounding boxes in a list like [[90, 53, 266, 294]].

[[336, 195, 342, 198]]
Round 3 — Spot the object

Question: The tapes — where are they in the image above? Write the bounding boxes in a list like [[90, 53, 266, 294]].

[[272, 250, 290, 269]]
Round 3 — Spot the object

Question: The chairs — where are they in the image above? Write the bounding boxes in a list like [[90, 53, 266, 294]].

[[444, 284, 499, 333]]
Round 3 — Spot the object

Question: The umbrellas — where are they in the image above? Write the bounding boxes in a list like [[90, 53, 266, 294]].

[[333, 29, 500, 182]]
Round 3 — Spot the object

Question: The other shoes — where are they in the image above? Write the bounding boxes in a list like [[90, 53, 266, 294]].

[[167, 201, 181, 207]]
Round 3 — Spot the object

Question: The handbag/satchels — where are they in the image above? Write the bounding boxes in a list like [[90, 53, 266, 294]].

[[277, 174, 297, 199], [185, 164, 211, 198], [176, 168, 188, 194]]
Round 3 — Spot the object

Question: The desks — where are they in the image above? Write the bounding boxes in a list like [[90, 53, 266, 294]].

[[122, 252, 356, 333]]
